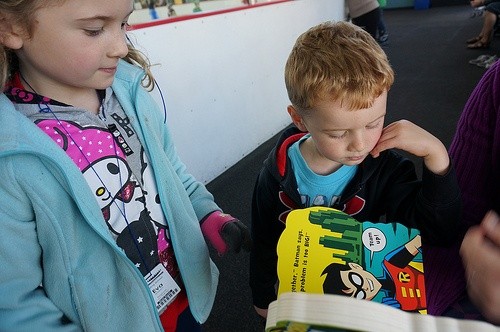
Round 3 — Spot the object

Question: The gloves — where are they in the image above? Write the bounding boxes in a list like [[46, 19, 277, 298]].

[[199, 209, 249, 260]]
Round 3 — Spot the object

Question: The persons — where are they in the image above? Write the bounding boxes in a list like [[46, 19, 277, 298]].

[[0, 0, 249, 332], [426, 0, 500, 331], [249, 20, 459, 332]]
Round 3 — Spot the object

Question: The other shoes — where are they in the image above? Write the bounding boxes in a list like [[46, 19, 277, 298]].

[[466, 35, 488, 49], [469, 55, 498, 68], [379, 33, 390, 44]]
